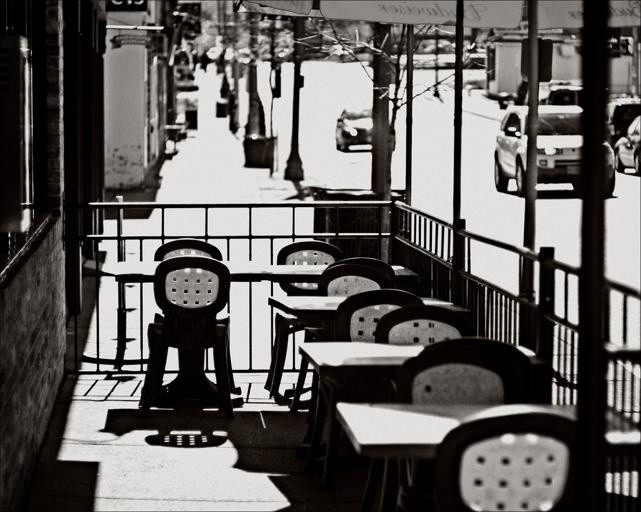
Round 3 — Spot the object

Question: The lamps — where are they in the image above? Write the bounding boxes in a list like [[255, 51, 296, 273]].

[[303, 0, 328, 37]]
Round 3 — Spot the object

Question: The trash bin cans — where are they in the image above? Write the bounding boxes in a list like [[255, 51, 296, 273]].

[[309, 186, 405, 260]]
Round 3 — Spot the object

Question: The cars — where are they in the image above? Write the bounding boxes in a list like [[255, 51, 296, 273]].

[[344, 34, 488, 70], [335, 108, 396, 155], [492, 77, 640, 199]]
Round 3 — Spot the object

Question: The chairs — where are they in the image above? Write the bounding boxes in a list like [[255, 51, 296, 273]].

[[428, 412, 609, 511], [265, 241, 344, 400], [153, 237, 243, 394], [331, 287, 426, 346], [139, 257, 236, 417], [376, 305, 476, 353], [280, 257, 396, 426], [403, 336, 541, 409]]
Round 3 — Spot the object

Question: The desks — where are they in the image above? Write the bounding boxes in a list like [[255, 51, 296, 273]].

[[98, 254, 418, 392], [265, 289, 458, 414], [332, 392, 639, 509], [292, 331, 542, 489]]
